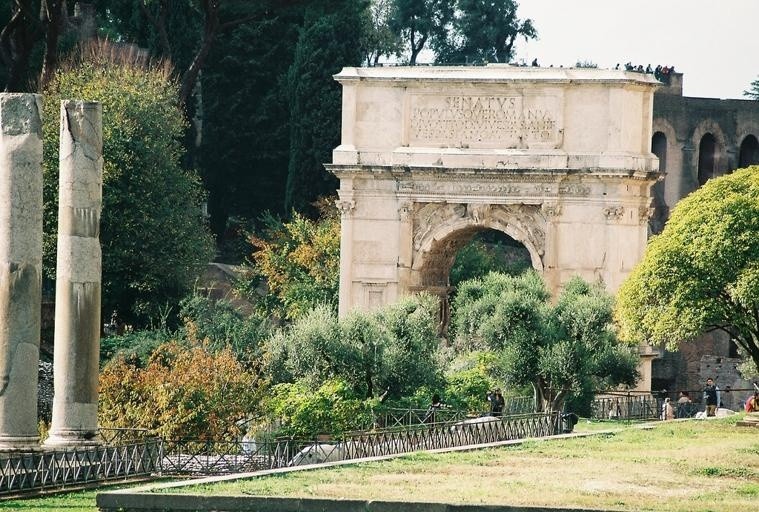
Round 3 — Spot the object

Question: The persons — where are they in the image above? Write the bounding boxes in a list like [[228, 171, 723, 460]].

[[745, 390, 759, 412], [704, 378, 720, 417], [487, 388, 505, 417], [662, 391, 691, 421], [426, 394, 453, 422]]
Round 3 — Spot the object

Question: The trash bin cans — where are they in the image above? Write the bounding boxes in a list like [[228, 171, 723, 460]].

[[562, 414, 573, 432]]
